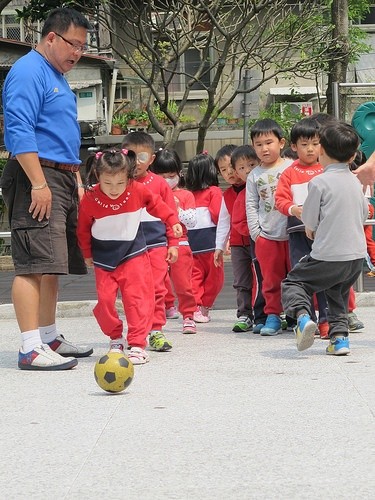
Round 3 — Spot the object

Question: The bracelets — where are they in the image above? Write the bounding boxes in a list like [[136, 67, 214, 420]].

[[77, 184, 85, 189], [31, 183, 48, 191]]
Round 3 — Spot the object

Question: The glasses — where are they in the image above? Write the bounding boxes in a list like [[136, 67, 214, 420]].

[[134, 152, 153, 163], [54, 31, 90, 55]]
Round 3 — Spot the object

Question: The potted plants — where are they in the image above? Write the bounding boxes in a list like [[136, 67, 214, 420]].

[[111, 103, 239, 135]]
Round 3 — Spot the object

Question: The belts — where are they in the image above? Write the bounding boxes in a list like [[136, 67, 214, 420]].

[[9, 153, 81, 174]]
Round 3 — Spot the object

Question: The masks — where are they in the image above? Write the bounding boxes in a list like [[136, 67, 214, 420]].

[[165, 174, 180, 189]]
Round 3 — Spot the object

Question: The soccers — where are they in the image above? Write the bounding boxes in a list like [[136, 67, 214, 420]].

[[94, 351, 135, 393]]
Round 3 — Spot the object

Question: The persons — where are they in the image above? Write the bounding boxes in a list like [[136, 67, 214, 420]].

[[350, 149, 375, 202], [304, 113, 365, 331], [279, 124, 367, 356], [245, 120, 320, 338], [347, 148, 375, 277], [163, 269, 180, 321], [212, 143, 255, 334], [273, 118, 331, 340], [77, 147, 180, 367], [183, 152, 226, 325], [123, 129, 179, 355], [0, 8, 94, 372], [148, 149, 196, 335], [230, 146, 300, 336]]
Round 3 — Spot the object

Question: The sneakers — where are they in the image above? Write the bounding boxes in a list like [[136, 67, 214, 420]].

[[149, 331, 172, 352], [108, 336, 126, 350], [183, 317, 196, 333], [192, 310, 209, 323], [233, 311, 365, 339], [124, 345, 150, 365], [325, 337, 350, 355], [198, 305, 211, 320], [19, 344, 78, 371], [165, 305, 179, 318], [47, 334, 93, 358], [292, 314, 317, 351]]
